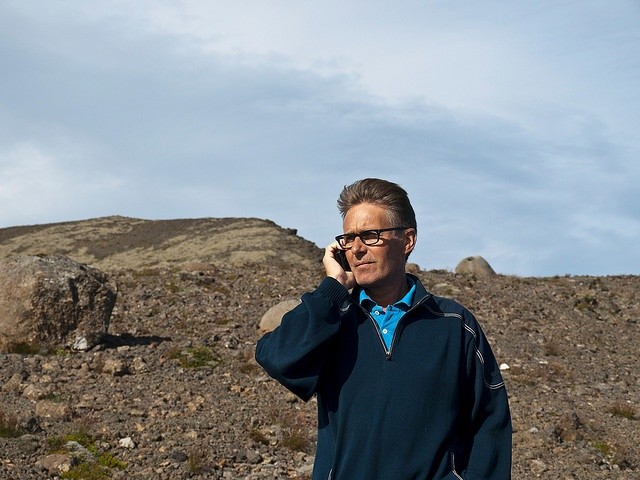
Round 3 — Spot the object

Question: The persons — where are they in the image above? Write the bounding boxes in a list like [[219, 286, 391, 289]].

[[255, 176, 512, 479]]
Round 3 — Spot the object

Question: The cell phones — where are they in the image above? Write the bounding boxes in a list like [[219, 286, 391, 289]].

[[333, 250, 351, 271]]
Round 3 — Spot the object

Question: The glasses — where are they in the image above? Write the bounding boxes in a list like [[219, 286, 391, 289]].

[[335, 226, 415, 249]]
